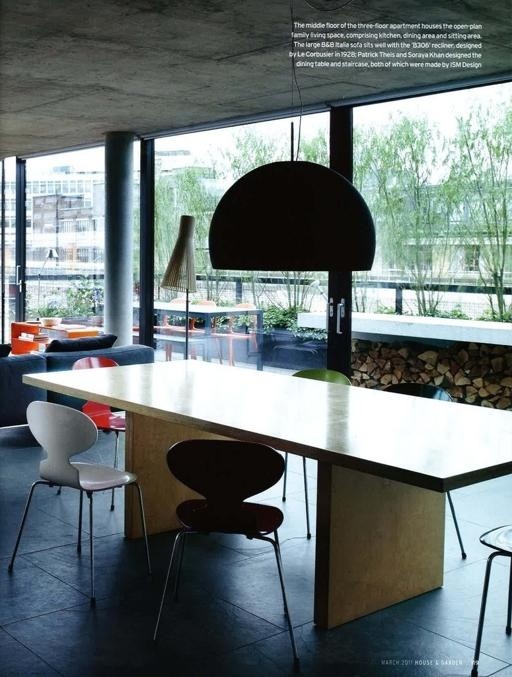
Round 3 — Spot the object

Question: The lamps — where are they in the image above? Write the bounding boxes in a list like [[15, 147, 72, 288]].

[[159, 214, 196, 361], [209, 0, 376, 270], [36, 248, 59, 324]]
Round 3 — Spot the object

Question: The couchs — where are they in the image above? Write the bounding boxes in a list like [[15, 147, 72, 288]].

[[0, 343, 156, 427]]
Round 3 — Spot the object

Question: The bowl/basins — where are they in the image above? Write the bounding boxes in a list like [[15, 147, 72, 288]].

[[40, 317, 63, 327]]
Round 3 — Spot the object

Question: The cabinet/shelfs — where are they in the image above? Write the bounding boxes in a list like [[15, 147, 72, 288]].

[[10, 321, 98, 355]]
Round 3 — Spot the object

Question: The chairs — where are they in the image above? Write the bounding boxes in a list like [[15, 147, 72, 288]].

[[133, 296, 264, 371]]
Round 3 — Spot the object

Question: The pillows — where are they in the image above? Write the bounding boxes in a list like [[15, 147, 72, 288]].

[[45, 333, 117, 352]]
